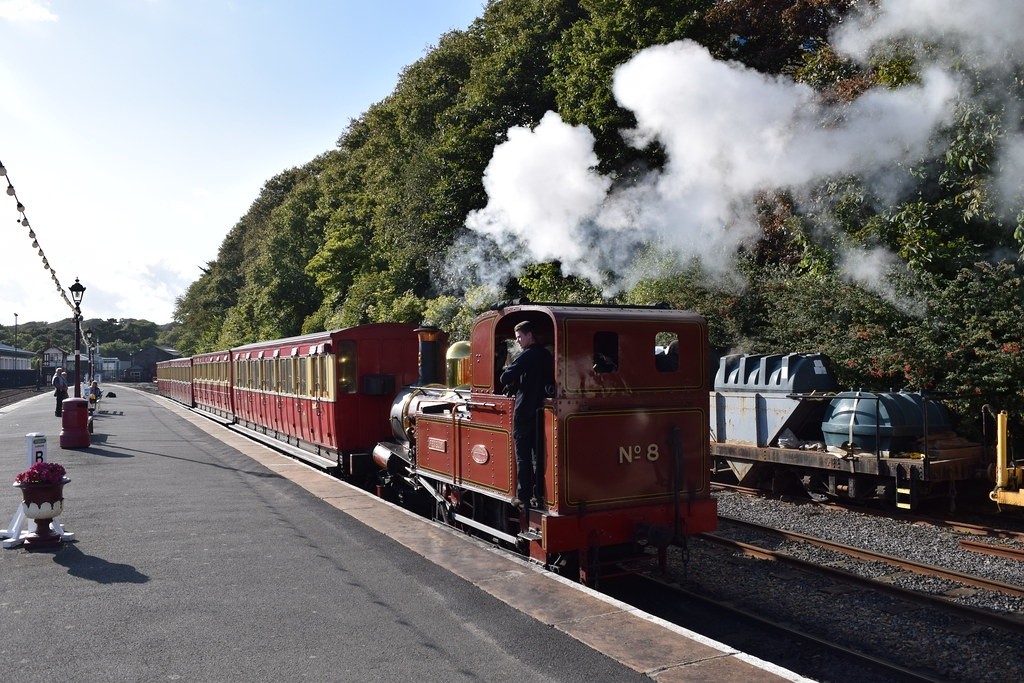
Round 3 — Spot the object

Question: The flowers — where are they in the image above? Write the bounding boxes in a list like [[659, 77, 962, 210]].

[[15, 462, 66, 485]]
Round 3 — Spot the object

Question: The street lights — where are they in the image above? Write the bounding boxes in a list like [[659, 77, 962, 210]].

[[85, 328, 92, 386], [67, 277, 86, 400], [13, 312, 18, 387], [90, 343, 96, 382]]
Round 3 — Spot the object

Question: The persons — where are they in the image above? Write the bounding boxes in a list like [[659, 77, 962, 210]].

[[52, 367, 70, 416], [89, 380, 102, 415], [500, 319, 555, 512]]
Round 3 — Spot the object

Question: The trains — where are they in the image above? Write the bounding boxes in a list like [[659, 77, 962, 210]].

[[154, 303, 720, 593], [706, 354, 988, 523]]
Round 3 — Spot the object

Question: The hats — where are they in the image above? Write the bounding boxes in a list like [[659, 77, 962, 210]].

[[61, 372, 67, 375]]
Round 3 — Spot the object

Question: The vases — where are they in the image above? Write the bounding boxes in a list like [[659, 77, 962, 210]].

[[13, 477, 72, 550]]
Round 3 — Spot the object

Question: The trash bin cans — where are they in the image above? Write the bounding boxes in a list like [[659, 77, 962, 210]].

[[59, 397, 91, 448]]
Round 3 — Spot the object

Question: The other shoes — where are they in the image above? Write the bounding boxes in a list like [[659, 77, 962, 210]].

[[530, 497, 543, 509], [511, 496, 530, 510]]
